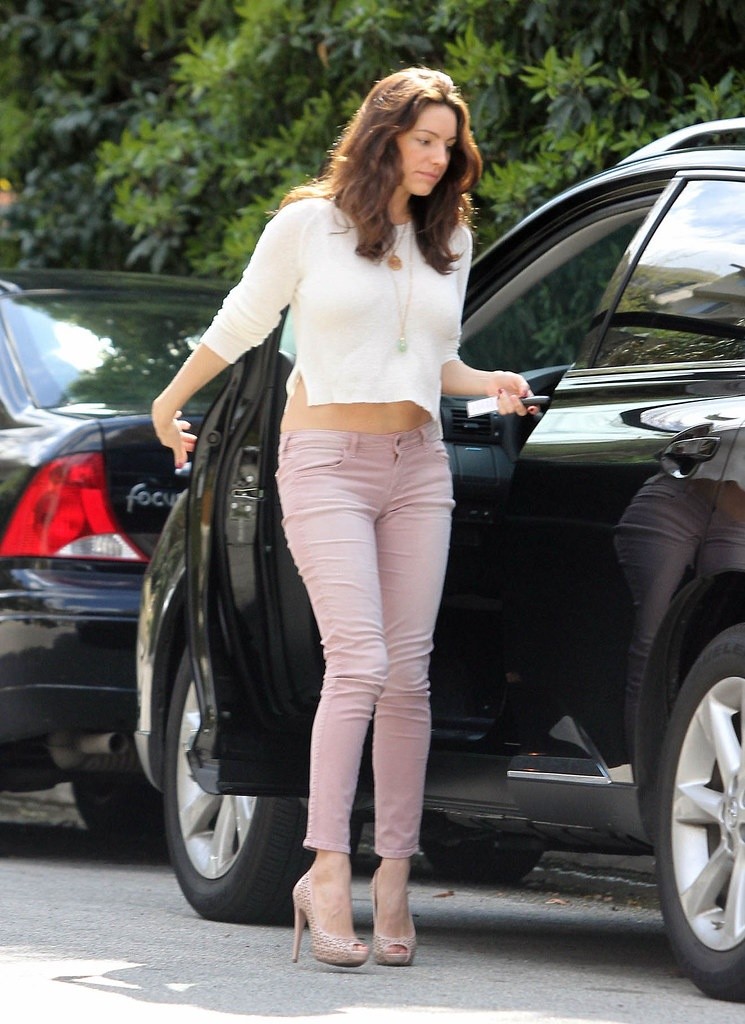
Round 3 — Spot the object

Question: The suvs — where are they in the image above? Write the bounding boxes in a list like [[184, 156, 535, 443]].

[[123, 116, 745, 1004]]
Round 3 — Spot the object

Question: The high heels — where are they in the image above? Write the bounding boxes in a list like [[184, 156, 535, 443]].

[[370, 867, 418, 967], [291, 871, 370, 968]]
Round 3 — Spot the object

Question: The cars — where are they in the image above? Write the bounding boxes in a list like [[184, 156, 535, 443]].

[[0, 270, 236, 856]]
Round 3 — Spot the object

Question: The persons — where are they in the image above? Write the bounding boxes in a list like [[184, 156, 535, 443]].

[[147, 64, 540, 969]]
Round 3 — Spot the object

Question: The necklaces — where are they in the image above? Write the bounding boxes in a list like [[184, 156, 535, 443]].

[[383, 215, 409, 272]]
